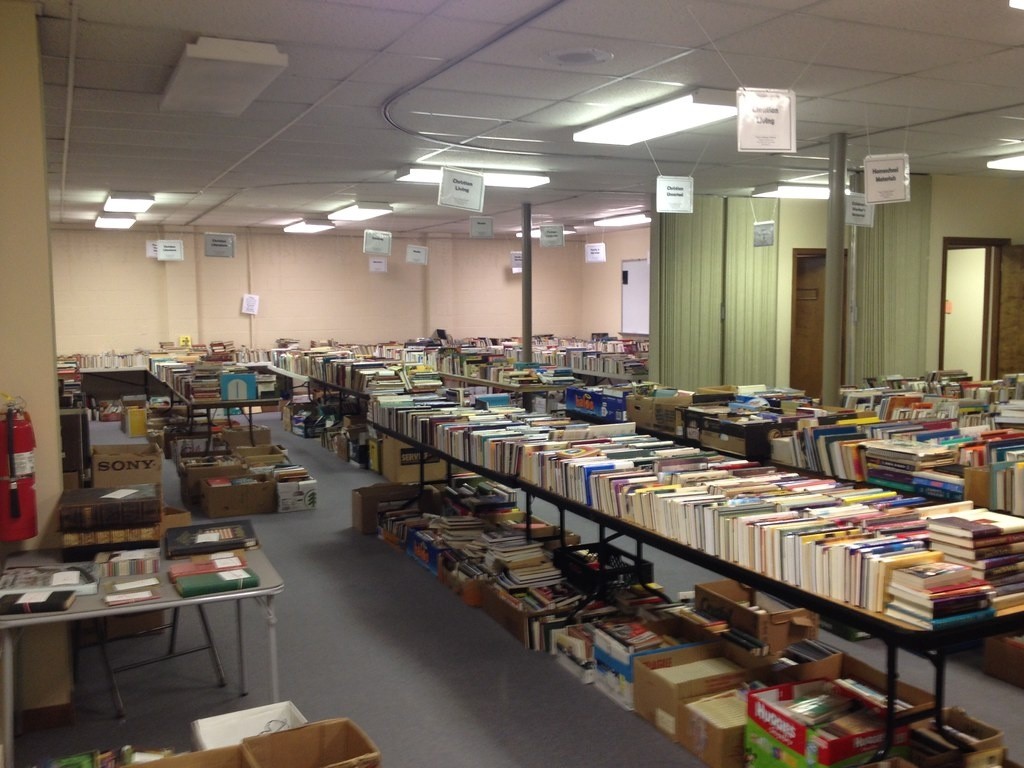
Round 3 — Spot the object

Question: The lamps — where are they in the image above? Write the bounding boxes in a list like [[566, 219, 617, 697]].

[[103, 191, 155, 213], [327, 202, 394, 222], [159, 37, 288, 118], [572, 87, 737, 147], [94, 212, 136, 230], [283, 220, 335, 234], [751, 183, 851, 200], [393, 165, 550, 189]]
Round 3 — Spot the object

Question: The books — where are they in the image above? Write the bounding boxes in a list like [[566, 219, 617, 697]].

[[0, 333, 1024, 768], [165, 516, 259, 556]]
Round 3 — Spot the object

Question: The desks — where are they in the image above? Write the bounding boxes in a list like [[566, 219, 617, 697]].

[[78, 367, 148, 394], [0, 544, 284, 719], [242, 362, 271, 368], [366, 418, 516, 505], [268, 364, 311, 401], [516, 475, 1024, 764], [571, 369, 650, 385], [565, 406, 699, 450], [146, 369, 184, 415], [165, 384, 279, 454], [438, 371, 568, 415], [307, 376, 370, 418]]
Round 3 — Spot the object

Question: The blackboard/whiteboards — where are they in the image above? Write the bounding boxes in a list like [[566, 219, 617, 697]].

[[618, 258, 650, 336]]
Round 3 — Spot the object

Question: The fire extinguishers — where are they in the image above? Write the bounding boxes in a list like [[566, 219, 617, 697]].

[[0, 391, 39, 542]]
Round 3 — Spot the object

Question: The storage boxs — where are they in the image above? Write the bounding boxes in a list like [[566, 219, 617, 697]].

[[242, 718, 382, 768], [189, 699, 310, 751], [60, 399, 322, 646], [323, 380, 1024, 768], [118, 744, 258, 768]]
